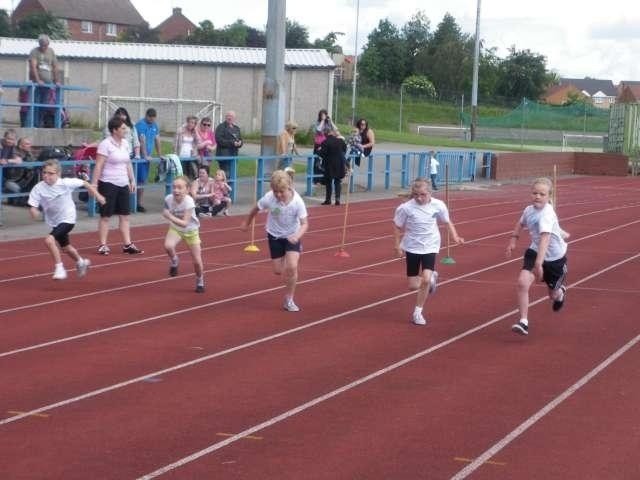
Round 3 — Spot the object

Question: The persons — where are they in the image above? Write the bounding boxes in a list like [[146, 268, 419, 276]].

[[163, 175, 205, 293], [79, 106, 162, 212], [176, 110, 244, 217], [320, 127, 347, 205], [91, 118, 145, 255], [504, 178, 571, 336], [27, 159, 106, 280], [28, 34, 60, 127], [238, 167, 310, 312], [278, 121, 302, 170], [313, 109, 375, 185], [390, 177, 464, 325], [1, 127, 37, 207], [427, 151, 440, 190]]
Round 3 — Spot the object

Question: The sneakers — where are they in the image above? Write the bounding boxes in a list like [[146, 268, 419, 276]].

[[52, 264, 67, 279], [76, 256, 91, 277], [412, 311, 426, 325], [198, 212, 212, 218], [511, 321, 529, 336], [97, 244, 110, 256], [170, 256, 180, 277], [121, 243, 144, 255], [430, 271, 438, 296], [552, 285, 566, 312], [195, 278, 205, 293], [283, 299, 300, 312]]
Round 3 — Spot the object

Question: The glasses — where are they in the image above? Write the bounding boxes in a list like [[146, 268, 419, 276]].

[[203, 124, 210, 126]]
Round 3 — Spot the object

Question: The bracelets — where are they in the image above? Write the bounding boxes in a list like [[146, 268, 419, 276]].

[[510, 234, 520, 241], [130, 178, 134, 184], [92, 184, 98, 187]]
